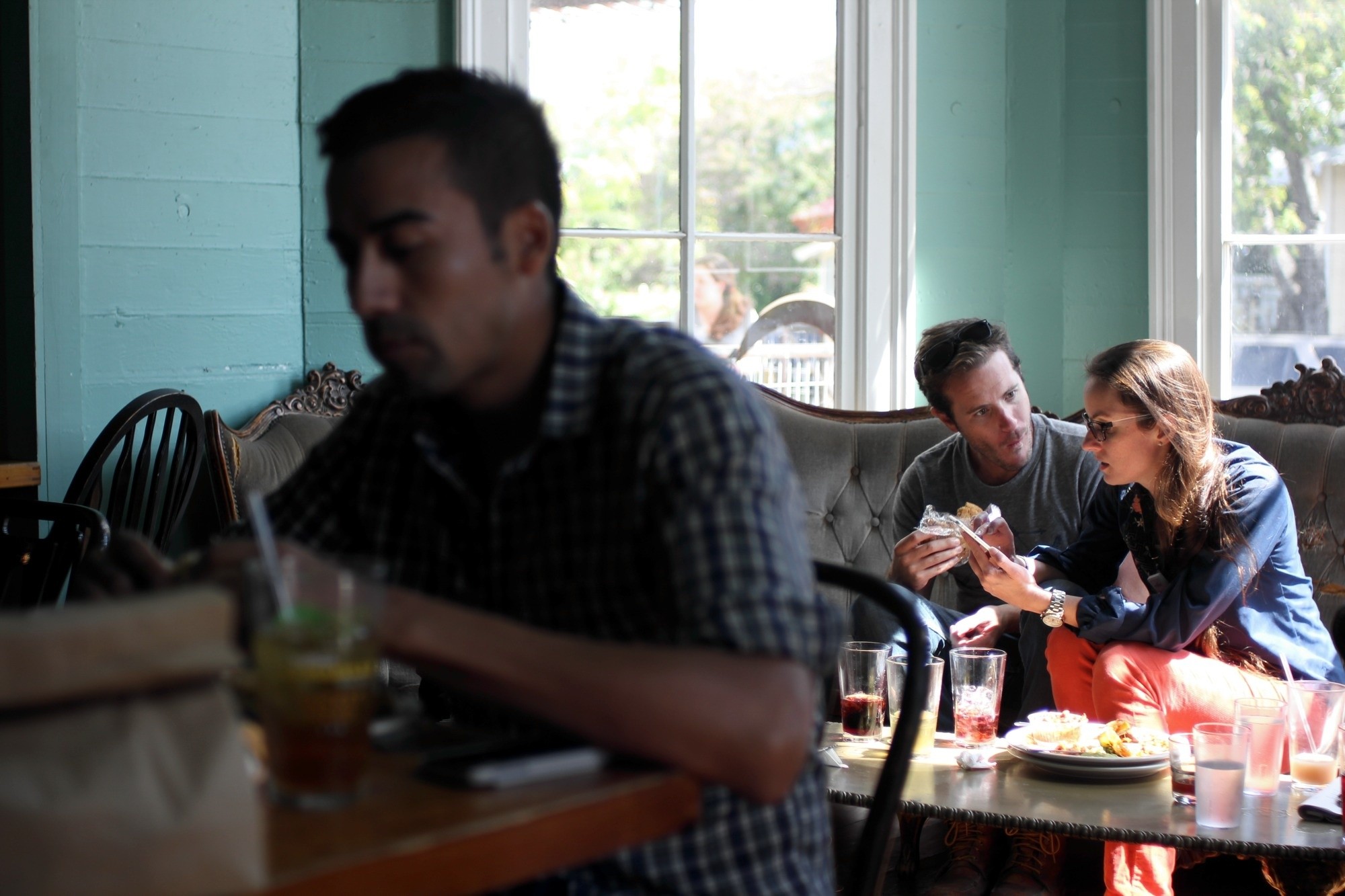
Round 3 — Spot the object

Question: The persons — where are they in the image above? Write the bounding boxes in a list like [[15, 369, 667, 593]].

[[85, 61, 841, 896], [851, 320, 1135, 750], [936, 336, 1344, 896]]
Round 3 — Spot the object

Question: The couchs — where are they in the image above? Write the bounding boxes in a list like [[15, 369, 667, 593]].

[[752, 353, 1345, 896], [219, 363, 381, 505]]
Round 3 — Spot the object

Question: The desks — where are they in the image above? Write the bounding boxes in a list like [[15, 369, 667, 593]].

[[278, 688, 704, 886]]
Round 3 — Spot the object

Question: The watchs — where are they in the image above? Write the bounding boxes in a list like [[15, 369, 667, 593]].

[[1040, 585, 1068, 630]]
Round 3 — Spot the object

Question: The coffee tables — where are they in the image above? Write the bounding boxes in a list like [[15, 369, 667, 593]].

[[805, 707, 1345, 896]]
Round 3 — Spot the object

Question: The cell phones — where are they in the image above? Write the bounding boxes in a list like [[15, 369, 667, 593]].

[[949, 517, 991, 552], [106, 531, 174, 589]]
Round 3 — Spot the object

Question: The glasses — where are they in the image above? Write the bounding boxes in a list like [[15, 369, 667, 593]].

[[1082, 413, 1152, 441]]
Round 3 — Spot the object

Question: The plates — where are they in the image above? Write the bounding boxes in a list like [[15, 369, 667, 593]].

[[1006, 722, 1177, 781]]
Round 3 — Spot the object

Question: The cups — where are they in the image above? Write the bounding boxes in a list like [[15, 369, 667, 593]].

[[837, 640, 892, 742], [950, 647, 1007, 752], [1168, 732, 1199, 807], [885, 654, 946, 758], [1232, 696, 1289, 796], [1192, 721, 1253, 828], [1284, 679, 1345, 793], [240, 553, 390, 807]]
